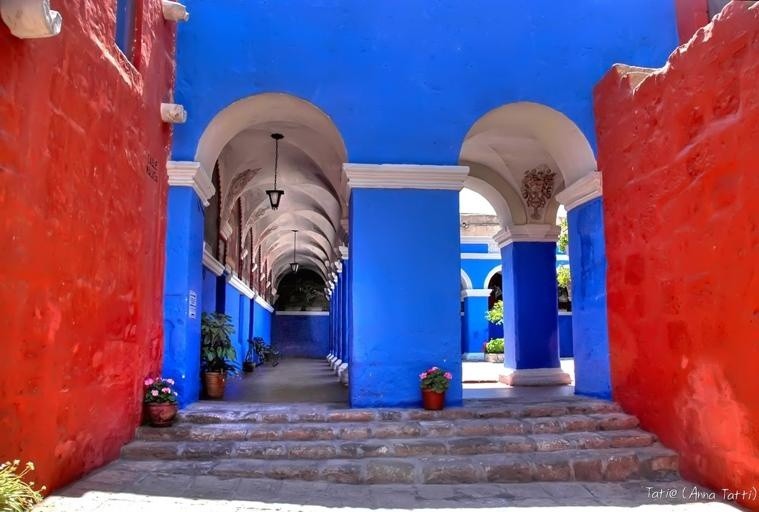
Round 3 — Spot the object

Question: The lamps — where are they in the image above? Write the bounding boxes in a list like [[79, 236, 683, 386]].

[[264, 133, 285, 210], [289, 229, 300, 273]]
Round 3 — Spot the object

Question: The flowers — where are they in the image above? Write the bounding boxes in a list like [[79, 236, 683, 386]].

[[418, 365, 453, 393], [142, 377, 179, 403]]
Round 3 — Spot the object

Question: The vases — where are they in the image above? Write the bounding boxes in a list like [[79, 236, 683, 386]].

[[423, 389, 446, 411], [148, 403, 178, 427]]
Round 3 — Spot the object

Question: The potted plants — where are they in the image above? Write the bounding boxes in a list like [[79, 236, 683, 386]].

[[201, 311, 238, 399], [485, 337, 505, 363], [243, 336, 281, 372]]
